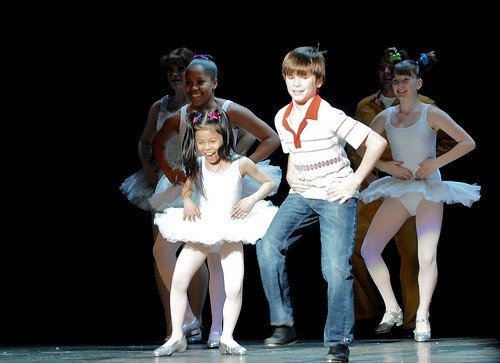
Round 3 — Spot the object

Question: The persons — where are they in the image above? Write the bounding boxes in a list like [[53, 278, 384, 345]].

[[121, 47, 480, 354], [255, 46, 388, 363]]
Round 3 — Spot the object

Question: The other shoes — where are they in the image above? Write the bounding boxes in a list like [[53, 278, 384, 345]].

[[218, 338, 247, 356], [376, 309, 403, 333], [186, 335, 203, 349], [324, 343, 350, 363], [413, 319, 431, 342], [153, 335, 188, 357], [164, 317, 199, 340], [207, 332, 222, 348], [264, 325, 298, 347]]
